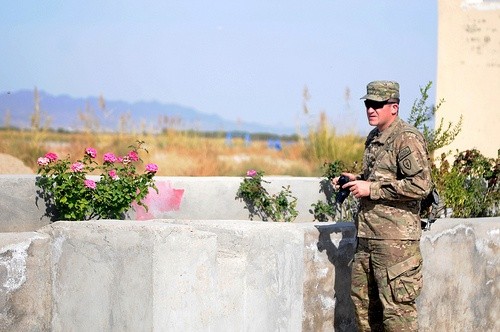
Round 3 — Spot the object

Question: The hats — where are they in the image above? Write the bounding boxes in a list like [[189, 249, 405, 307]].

[[359, 81, 400, 102]]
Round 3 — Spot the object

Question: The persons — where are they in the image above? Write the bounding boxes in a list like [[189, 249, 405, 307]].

[[330, 80, 433, 332]]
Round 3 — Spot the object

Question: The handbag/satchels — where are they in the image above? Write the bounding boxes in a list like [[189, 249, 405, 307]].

[[368, 128, 439, 231]]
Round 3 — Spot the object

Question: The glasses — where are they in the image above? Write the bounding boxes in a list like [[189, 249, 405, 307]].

[[364, 99, 397, 109]]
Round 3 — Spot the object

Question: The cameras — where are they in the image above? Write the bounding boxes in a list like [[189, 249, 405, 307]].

[[335, 175, 353, 204]]
